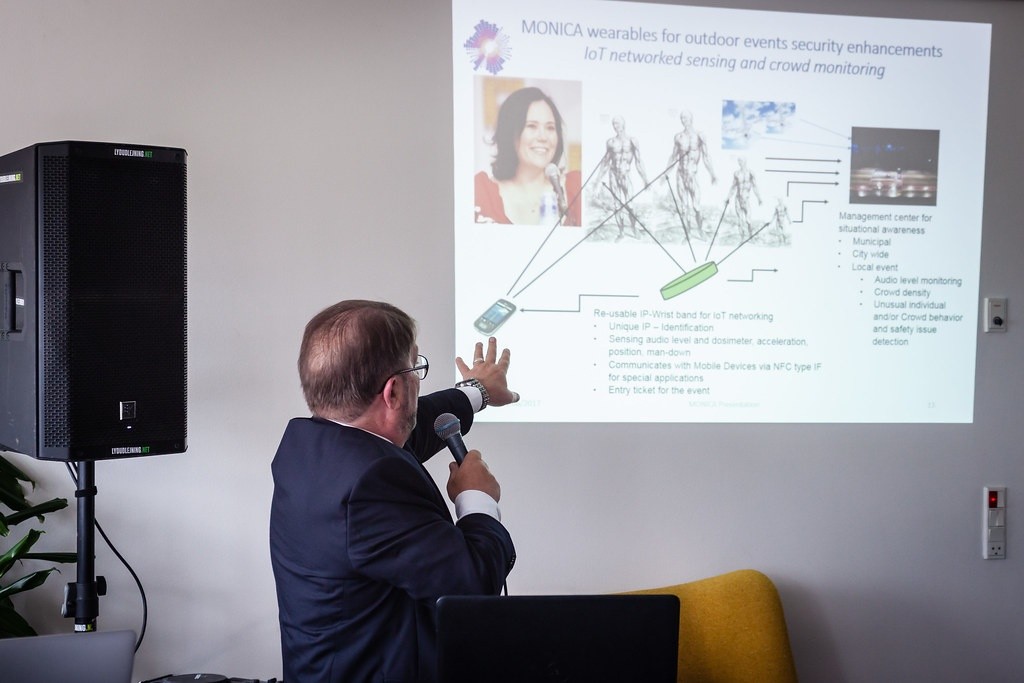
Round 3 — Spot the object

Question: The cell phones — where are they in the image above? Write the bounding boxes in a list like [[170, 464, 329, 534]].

[[473, 299, 516, 336]]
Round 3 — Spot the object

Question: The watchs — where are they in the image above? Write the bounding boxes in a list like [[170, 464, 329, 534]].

[[455, 378, 489, 412]]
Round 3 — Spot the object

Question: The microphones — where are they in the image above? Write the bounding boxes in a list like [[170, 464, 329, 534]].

[[434, 414, 469, 468], [544, 162, 568, 215]]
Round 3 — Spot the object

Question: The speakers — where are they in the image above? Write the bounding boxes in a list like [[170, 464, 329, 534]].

[[0, 140, 189, 461]]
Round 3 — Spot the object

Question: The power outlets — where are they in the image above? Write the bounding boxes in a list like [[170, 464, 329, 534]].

[[983, 487, 1007, 559]]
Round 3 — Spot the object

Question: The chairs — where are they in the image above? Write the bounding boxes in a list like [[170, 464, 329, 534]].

[[615, 570, 794, 683]]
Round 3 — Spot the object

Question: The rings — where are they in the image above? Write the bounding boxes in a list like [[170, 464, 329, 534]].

[[472, 358, 484, 364]]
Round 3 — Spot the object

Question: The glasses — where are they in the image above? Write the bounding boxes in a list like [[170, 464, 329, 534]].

[[377, 355, 429, 395]]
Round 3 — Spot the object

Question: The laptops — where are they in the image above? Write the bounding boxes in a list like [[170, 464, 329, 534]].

[[0, 629, 138, 683], [435, 594, 680, 683]]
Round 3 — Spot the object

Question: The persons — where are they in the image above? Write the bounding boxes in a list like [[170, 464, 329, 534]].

[[474, 87, 575, 225], [270, 300, 520, 683]]
